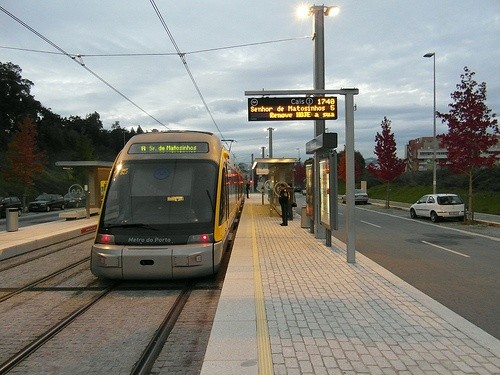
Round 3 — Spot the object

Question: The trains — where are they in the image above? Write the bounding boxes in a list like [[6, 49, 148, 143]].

[[89, 129, 244, 281]]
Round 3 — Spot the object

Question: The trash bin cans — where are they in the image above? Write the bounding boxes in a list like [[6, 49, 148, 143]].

[[5, 207, 19, 232], [299, 205, 311, 228]]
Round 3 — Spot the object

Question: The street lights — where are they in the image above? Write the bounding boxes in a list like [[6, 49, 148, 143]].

[[264, 127, 277, 158], [423, 52, 437, 193], [297, 4, 342, 136], [259, 146, 267, 158]]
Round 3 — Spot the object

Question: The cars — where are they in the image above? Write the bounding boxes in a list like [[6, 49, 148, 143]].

[[409, 193, 465, 222], [341, 189, 368, 204], [0, 196, 23, 217], [28, 194, 65, 212], [64, 192, 87, 208]]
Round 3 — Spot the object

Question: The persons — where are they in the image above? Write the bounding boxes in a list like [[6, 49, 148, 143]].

[[246, 180, 251, 199], [279, 184, 288, 226]]
